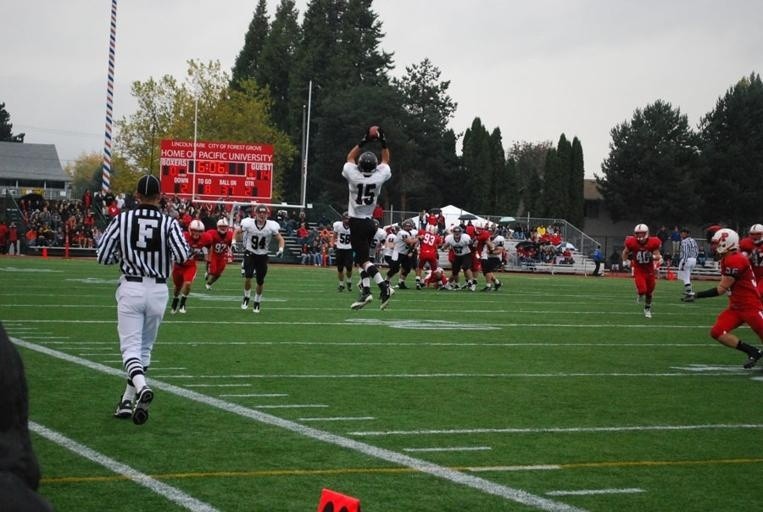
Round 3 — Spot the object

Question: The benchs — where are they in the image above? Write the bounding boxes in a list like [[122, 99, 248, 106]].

[[189, 244, 274, 258], [414, 234, 600, 269], [274, 219, 357, 261]]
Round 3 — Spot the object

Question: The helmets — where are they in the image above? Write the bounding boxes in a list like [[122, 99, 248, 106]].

[[634, 224, 649, 242], [189, 220, 204, 236], [712, 228, 739, 254], [750, 224, 763, 245], [217, 220, 228, 234], [255, 205, 268, 212], [358, 152, 378, 173]]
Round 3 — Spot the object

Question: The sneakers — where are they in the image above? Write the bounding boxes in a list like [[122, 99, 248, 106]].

[[415, 278, 425, 290], [743, 348, 762, 369], [377, 280, 396, 310], [253, 301, 260, 313], [241, 297, 250, 310], [644, 307, 652, 318], [439, 278, 502, 292], [171, 296, 187, 314], [114, 395, 132, 418], [131, 386, 154, 425], [351, 282, 373, 310]]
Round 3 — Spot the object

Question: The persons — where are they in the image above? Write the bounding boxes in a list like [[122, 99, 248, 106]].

[[0, 190, 140, 257], [657, 225, 682, 267], [341, 128, 394, 309], [232, 204, 285, 313], [622, 223, 663, 317], [169, 220, 207, 314], [741, 223, 762, 275], [203, 218, 234, 289], [492, 222, 575, 265], [159, 191, 254, 232], [279, 212, 336, 267], [592, 246, 606, 276], [678, 230, 698, 301], [356, 212, 505, 293], [332, 212, 354, 291], [96, 174, 190, 425], [681, 228, 763, 369]]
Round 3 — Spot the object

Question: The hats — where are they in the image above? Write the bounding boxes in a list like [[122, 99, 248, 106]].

[[137, 174, 160, 195]]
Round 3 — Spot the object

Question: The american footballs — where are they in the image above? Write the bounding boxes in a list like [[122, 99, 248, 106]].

[[369, 126, 379, 141]]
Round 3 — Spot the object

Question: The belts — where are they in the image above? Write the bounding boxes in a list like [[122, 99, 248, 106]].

[[125, 276, 166, 283]]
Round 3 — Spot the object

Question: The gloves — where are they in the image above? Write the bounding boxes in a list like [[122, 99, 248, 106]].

[[681, 292, 696, 302], [276, 248, 284, 259], [231, 242, 240, 253]]
